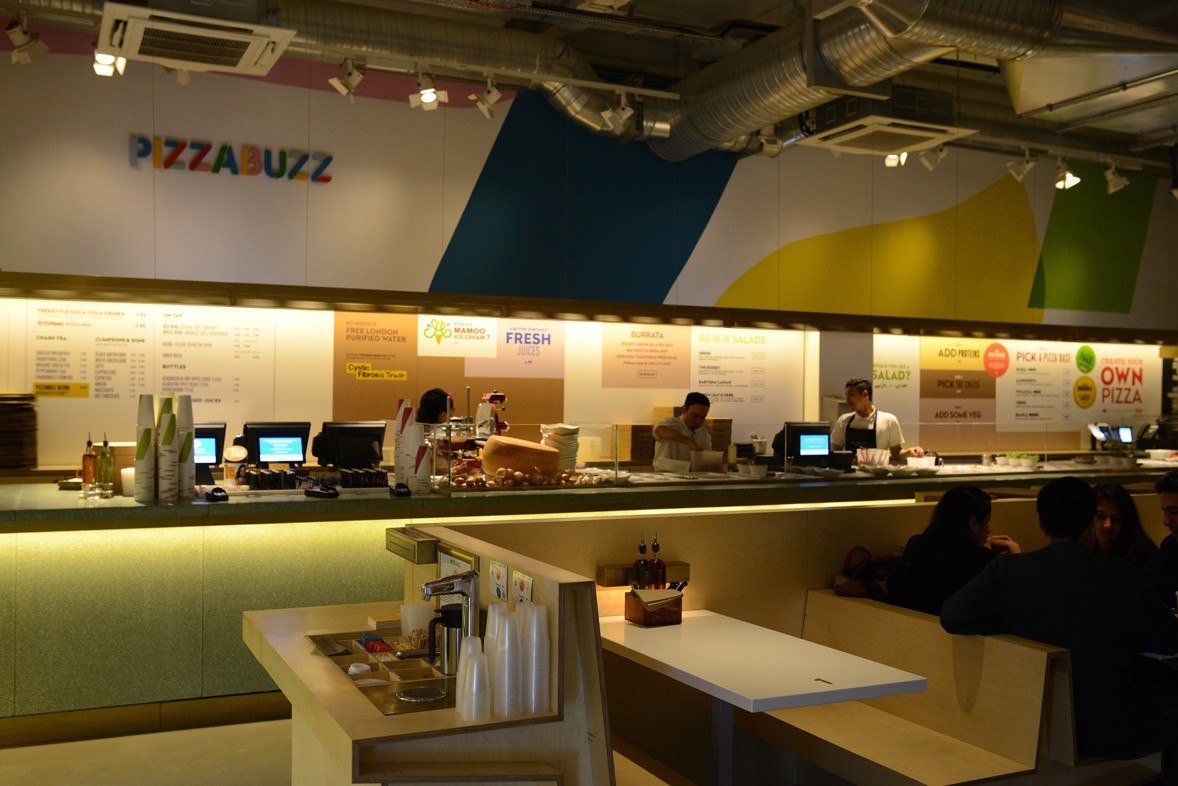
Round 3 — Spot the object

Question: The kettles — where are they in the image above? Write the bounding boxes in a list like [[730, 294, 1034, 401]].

[[429, 602, 462, 678]]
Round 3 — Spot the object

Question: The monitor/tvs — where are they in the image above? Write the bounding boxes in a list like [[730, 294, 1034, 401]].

[[241, 423, 311, 467], [191, 422, 227, 485], [783, 422, 832, 469], [319, 422, 385, 467], [1116, 426, 1132, 444]]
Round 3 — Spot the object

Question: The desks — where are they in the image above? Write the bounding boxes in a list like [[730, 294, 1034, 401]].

[[598, 609, 928, 786]]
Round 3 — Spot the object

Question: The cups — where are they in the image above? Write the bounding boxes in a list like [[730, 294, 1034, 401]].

[[394, 398, 432, 494], [134, 394, 196, 503], [455, 600, 550, 723]]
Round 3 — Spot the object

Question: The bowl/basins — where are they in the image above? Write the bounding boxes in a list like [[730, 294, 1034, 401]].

[[752, 439, 767, 454], [749, 464, 768, 478], [814, 468, 845, 477], [612, 473, 631, 485], [907, 457, 929, 468], [736, 463, 749, 475], [922, 456, 936, 468], [799, 466, 818, 475], [1144, 449, 1178, 460], [995, 457, 1036, 467], [690, 450, 723, 473]]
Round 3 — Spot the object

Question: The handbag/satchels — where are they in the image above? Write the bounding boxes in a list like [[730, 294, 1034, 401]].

[[833, 546, 898, 601]]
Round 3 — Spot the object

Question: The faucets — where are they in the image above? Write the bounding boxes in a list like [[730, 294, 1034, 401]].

[[420, 569, 479, 637]]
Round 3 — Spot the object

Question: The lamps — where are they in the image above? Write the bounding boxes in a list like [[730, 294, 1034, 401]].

[[599, 91, 635, 135], [1105, 159, 1132, 195], [94, 48, 127, 77], [409, 70, 449, 111], [1054, 156, 1081, 189], [467, 76, 502, 120], [1006, 148, 1037, 182], [5, 7, 50, 64], [920, 142, 950, 171], [327, 59, 367, 104], [163, 65, 193, 85]]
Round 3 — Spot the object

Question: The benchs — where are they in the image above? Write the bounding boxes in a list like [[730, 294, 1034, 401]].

[[612, 733, 699, 786], [732, 586, 1164, 786]]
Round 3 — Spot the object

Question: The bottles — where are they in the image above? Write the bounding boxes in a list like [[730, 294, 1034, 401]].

[[648, 531, 666, 590], [727, 439, 737, 472], [632, 532, 649, 589], [96, 432, 114, 499], [82, 431, 96, 492]]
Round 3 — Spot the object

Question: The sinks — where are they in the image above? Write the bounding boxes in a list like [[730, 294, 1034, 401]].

[[304, 625, 457, 714]]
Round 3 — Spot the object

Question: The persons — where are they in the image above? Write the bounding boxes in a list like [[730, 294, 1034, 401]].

[[1094, 471, 1178, 608], [653, 392, 712, 472], [831, 378, 924, 460], [903, 485, 1021, 615], [416, 388, 455, 473], [940, 478, 1178, 786]]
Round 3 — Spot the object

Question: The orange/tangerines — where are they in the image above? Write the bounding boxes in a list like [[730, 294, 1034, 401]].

[[436, 467, 574, 491]]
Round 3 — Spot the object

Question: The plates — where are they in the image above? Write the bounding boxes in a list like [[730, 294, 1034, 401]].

[[539, 423, 579, 474], [859, 464, 939, 477]]
